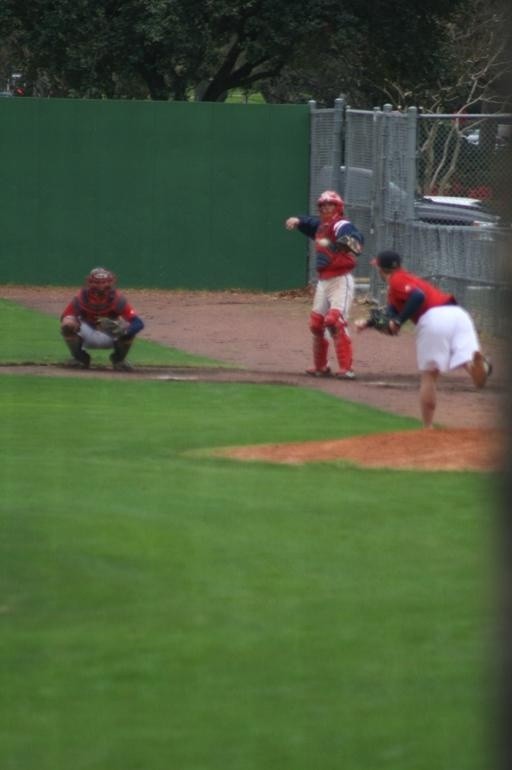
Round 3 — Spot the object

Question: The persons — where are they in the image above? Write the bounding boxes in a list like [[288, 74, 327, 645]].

[[285, 190, 370, 385], [57, 266, 144, 374], [355, 250, 491, 433]]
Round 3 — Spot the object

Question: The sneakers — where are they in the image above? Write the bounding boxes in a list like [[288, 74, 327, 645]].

[[305, 367, 330, 377], [336, 366, 355, 379], [471, 352, 491, 387], [66, 350, 91, 369], [109, 353, 132, 371]]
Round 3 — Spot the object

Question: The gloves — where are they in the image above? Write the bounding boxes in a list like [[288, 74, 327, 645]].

[[112, 323, 128, 342]]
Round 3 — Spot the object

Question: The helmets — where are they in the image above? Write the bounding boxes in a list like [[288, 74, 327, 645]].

[[86, 267, 114, 290], [317, 190, 344, 220]]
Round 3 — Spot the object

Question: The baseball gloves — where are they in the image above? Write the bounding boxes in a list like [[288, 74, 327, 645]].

[[371, 308, 394, 336], [98, 319, 125, 337]]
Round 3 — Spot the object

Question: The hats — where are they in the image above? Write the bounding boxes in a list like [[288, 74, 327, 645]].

[[369, 251, 402, 270]]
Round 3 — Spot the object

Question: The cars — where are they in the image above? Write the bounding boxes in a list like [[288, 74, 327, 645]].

[[467, 123, 512, 148], [414, 195, 502, 227]]
